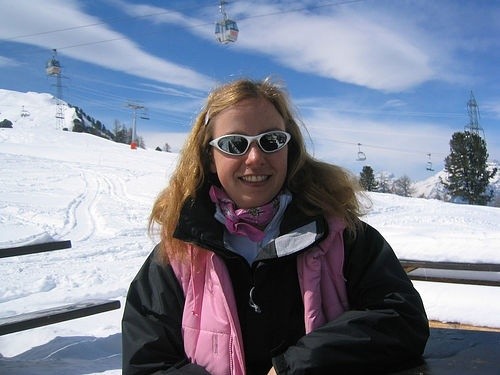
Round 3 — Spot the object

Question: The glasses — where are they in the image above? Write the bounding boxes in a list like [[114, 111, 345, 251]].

[[208, 131, 291, 156]]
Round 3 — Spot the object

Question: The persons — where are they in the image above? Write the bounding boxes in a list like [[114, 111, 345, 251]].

[[121, 80, 430, 375]]
